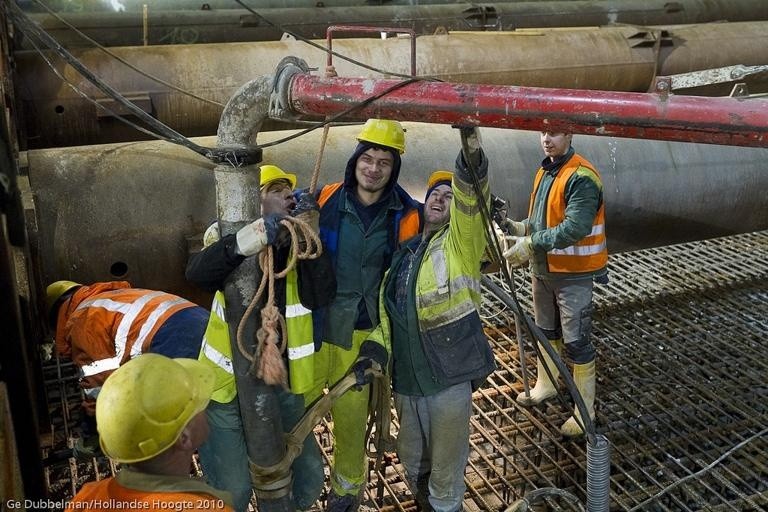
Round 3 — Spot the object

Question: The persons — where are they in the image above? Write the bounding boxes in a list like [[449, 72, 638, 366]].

[[497, 132, 609, 437], [39, 279, 213, 460], [378, 116, 498, 512], [63, 353, 234, 511], [297, 118, 425, 511], [185, 164, 338, 512]]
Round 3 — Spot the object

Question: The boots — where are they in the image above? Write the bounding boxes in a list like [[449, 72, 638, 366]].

[[517, 339, 561, 406], [561, 359, 596, 436]]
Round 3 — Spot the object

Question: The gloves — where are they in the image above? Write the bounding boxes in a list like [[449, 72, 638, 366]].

[[495, 217, 525, 236], [461, 124, 483, 166], [290, 195, 321, 240], [346, 356, 386, 392], [503, 236, 535, 268], [235, 212, 286, 257]]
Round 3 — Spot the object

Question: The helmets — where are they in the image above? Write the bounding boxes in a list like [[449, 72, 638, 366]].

[[356, 118, 405, 153], [428, 171, 454, 190], [95, 353, 216, 464], [44, 280, 82, 310], [259, 165, 297, 192]]
